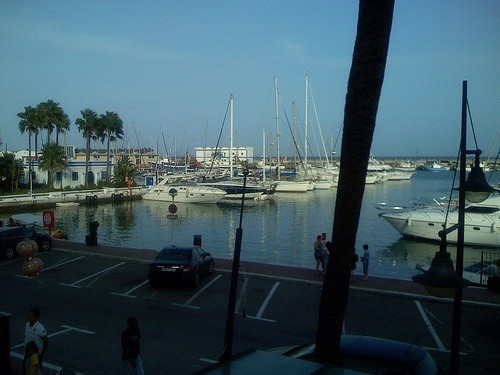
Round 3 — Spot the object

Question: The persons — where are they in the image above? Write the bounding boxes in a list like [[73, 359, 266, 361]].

[[350, 247, 358, 284], [6, 218, 18, 227], [119, 316, 145, 375], [321, 233, 328, 250], [0, 220, 4, 228], [325, 241, 332, 256], [360, 244, 370, 280], [313, 234, 327, 272], [22, 309, 48, 375], [23, 340, 41, 375], [55, 366, 82, 375]]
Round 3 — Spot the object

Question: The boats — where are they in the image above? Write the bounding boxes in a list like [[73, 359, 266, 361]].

[[375, 202, 402, 210], [416, 259, 500, 290], [382, 193, 499, 245], [265, 76, 416, 191], [198, 95, 268, 202], [141, 175, 227, 203], [416, 160, 490, 171]]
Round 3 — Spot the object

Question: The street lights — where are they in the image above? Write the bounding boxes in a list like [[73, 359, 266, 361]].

[[449, 80, 492, 374]]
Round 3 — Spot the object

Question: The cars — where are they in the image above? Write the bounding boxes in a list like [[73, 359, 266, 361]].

[[149, 245, 214, 288], [0, 227, 52, 260], [213, 335, 437, 375]]
